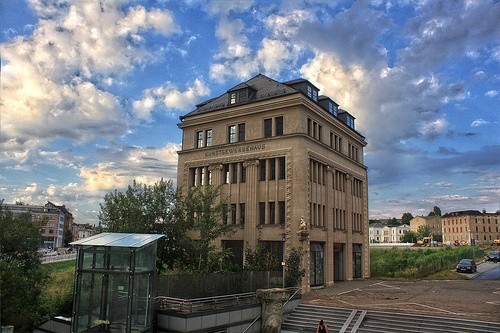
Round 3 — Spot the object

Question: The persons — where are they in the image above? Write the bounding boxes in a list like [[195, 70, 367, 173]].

[[317, 320, 328, 333]]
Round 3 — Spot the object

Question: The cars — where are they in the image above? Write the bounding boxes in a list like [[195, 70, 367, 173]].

[[486, 251, 500, 262], [456, 258, 477, 273]]
